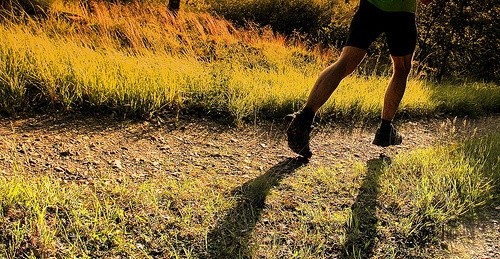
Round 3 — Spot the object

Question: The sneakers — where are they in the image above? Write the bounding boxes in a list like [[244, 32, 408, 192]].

[[373, 126, 402, 147], [287, 110, 312, 157]]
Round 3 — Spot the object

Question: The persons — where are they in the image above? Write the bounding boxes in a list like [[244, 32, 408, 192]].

[[287, 0, 417, 161]]
